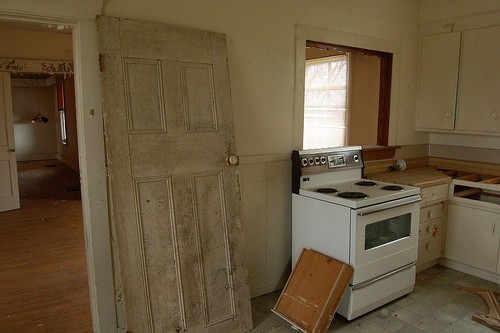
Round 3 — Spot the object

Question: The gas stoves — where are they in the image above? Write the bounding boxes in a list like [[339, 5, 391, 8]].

[[291, 145, 420, 210]]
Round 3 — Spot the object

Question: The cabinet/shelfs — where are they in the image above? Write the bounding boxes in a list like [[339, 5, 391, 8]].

[[441, 177, 500, 287], [413, 26, 499, 137], [367, 179, 452, 274]]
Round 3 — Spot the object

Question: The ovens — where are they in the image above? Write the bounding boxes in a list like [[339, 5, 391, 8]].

[[290, 193, 422, 321]]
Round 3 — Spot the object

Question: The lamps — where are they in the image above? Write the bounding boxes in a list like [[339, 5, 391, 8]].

[[29, 74, 50, 129]]
